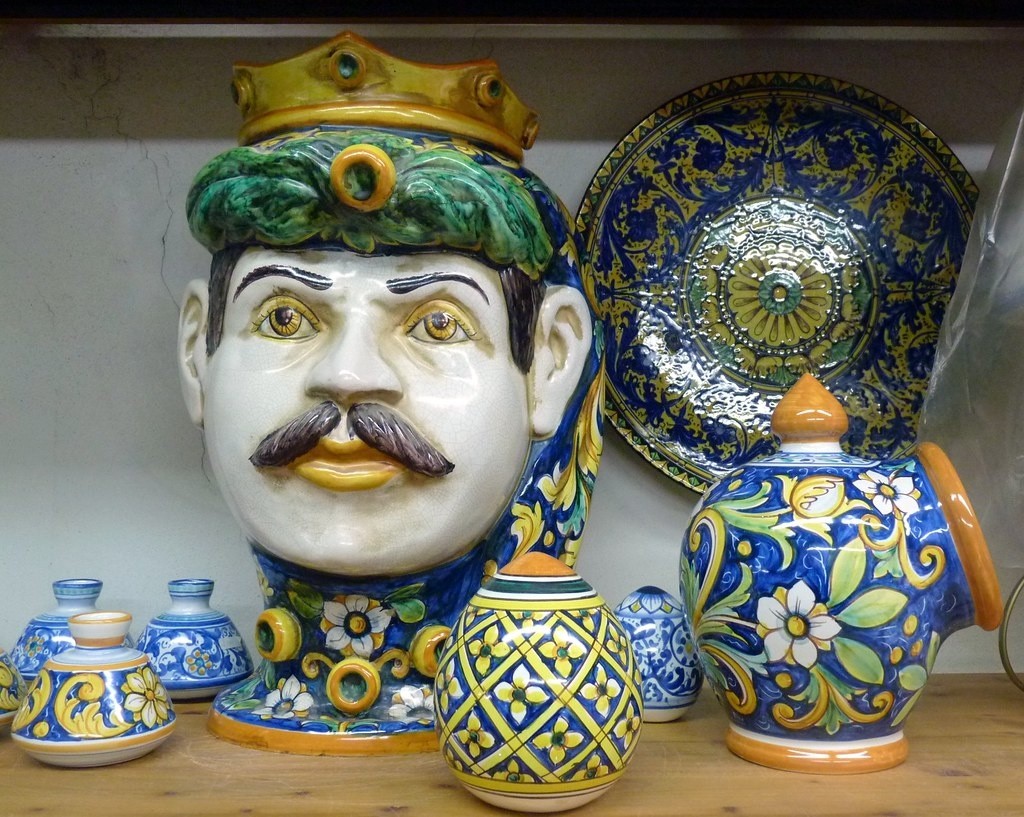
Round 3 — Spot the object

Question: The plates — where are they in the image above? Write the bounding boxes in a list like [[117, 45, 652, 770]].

[[573, 71, 980, 498]]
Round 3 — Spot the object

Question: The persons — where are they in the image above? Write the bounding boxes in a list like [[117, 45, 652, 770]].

[[176, 32, 607, 582]]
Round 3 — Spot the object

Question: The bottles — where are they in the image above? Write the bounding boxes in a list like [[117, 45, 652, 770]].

[[10, 579, 135, 689], [9, 612, 178, 770], [0, 646, 28, 730], [134, 578, 255, 698]]
